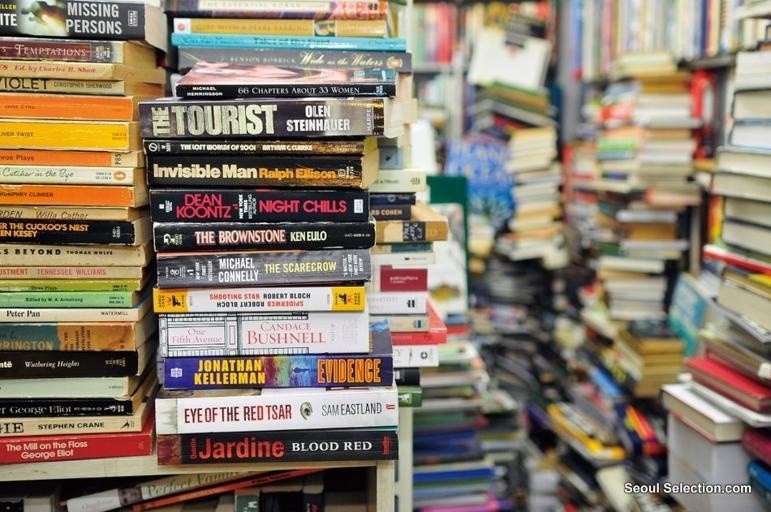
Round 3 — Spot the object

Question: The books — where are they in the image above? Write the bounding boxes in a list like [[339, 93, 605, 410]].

[[1, 470, 326, 512], [0, 0, 167, 463], [140, 0, 446, 463], [402, 0, 771, 512]]
